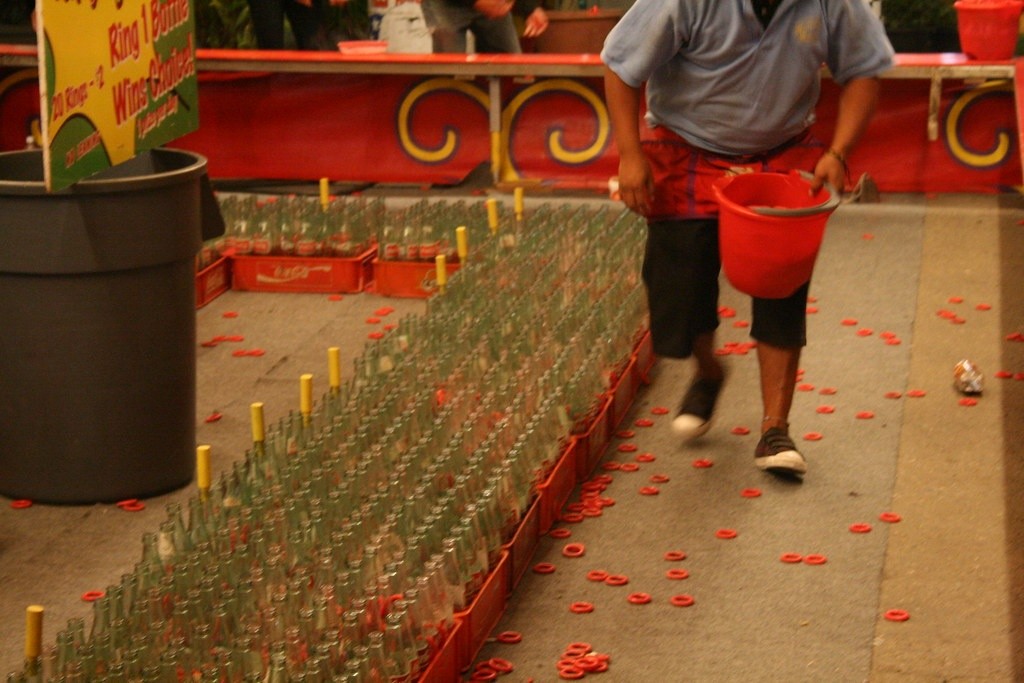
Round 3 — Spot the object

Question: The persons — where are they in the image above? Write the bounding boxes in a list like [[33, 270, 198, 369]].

[[248, 0, 371, 52], [598, 1, 896, 474], [421, 0, 549, 54]]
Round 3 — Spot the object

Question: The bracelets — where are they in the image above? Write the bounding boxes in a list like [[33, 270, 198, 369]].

[[827, 148, 853, 186]]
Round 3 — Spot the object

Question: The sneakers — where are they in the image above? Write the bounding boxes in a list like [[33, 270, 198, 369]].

[[754, 427, 807, 474], [673, 366, 725, 438]]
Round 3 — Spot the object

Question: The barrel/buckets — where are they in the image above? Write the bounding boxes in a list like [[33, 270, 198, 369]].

[[953, 0, 1024, 60], [2, 149, 209, 507], [714, 169, 841, 298]]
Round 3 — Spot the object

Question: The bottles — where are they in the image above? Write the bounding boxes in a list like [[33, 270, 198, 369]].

[[0, 191, 650, 683]]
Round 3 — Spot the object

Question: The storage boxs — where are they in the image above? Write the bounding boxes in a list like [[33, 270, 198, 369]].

[[417, 333, 660, 683], [195, 245, 468, 315]]
